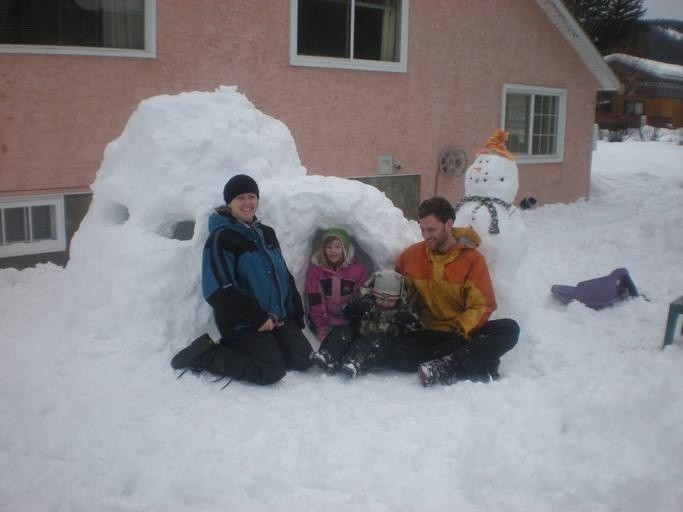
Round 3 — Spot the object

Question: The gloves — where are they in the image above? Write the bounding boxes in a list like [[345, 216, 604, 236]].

[[519, 197, 536, 210]]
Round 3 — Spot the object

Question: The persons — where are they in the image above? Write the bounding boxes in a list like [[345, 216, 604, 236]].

[[394, 195, 521, 388], [170, 173, 314, 387], [305, 227, 368, 343], [307, 268, 422, 380]]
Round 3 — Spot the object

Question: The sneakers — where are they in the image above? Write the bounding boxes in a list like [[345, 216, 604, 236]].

[[418, 359, 448, 387], [170, 333, 214, 379], [309, 351, 336, 375], [342, 363, 357, 379]]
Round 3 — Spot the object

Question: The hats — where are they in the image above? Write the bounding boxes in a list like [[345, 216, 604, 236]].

[[479, 130, 513, 161], [320, 229, 350, 265], [373, 270, 404, 300], [224, 175, 259, 204]]
